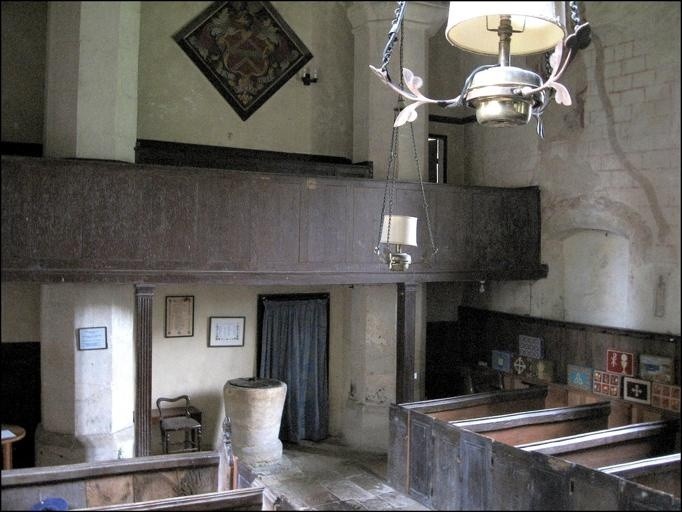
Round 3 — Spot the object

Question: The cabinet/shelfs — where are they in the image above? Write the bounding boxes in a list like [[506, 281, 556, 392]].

[[157, 394, 203, 455]]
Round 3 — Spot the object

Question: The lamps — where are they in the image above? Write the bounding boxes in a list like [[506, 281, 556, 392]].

[[366, 1, 593, 140], [372, 1, 449, 274]]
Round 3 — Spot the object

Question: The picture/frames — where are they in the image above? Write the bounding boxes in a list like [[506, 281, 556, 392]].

[[78, 326, 107, 350], [165, 295, 194, 338], [209, 317, 246, 346]]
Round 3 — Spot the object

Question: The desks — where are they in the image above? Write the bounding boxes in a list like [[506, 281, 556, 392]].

[[0, 424, 26, 470], [133, 406, 202, 452]]
